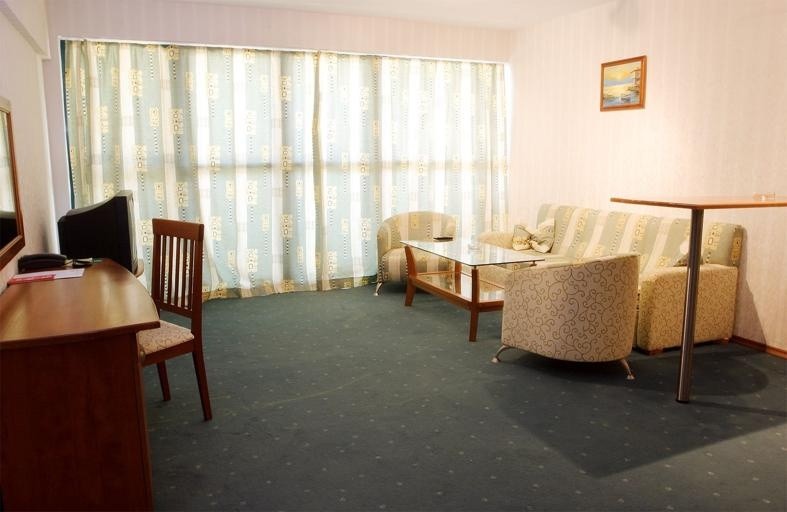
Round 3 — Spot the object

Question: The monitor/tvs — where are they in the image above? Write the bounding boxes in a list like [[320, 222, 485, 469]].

[[0, 210, 17, 251], [57, 190, 138, 276]]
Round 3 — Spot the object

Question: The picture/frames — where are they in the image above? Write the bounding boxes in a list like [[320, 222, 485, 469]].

[[600, 55, 648, 112]]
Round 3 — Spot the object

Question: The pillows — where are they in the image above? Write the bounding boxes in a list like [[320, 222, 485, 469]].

[[512, 218, 555, 254]]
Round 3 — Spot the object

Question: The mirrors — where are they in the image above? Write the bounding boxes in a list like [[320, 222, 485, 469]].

[[0, 96, 25, 271]]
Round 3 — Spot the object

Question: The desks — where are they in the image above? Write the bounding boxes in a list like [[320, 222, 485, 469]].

[[1, 257, 160, 512], [610, 197, 786, 404]]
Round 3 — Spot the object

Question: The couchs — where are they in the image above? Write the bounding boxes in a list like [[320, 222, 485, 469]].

[[492, 254, 642, 380], [374, 211, 457, 296], [478, 202, 744, 355]]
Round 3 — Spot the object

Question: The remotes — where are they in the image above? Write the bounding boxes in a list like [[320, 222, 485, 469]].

[[434, 236, 453, 240]]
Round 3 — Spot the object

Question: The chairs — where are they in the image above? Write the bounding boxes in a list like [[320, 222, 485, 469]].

[[136, 218, 212, 421]]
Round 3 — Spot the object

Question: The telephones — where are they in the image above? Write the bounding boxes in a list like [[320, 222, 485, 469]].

[[18, 254, 67, 274]]
